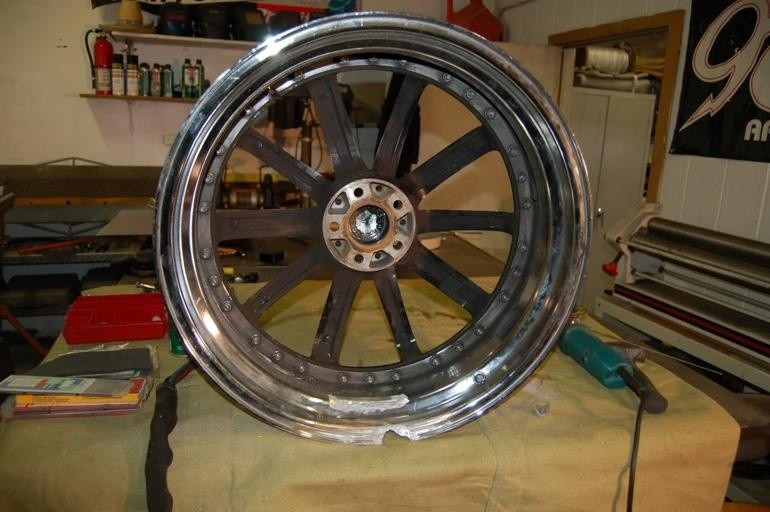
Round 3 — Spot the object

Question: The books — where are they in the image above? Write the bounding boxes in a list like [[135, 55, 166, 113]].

[[0, 371, 154, 414]]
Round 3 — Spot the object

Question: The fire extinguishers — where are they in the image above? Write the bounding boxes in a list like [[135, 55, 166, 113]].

[[85, 28, 117, 96]]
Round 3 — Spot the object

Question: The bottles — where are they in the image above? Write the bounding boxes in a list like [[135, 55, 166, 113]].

[[112, 55, 206, 98]]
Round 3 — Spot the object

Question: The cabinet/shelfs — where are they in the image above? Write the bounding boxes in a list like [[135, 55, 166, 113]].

[[570, 84, 658, 314], [79, 30, 262, 109], [0, 166, 315, 320]]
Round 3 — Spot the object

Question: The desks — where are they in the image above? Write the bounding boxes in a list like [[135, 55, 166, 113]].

[[0, 232, 770, 511]]
